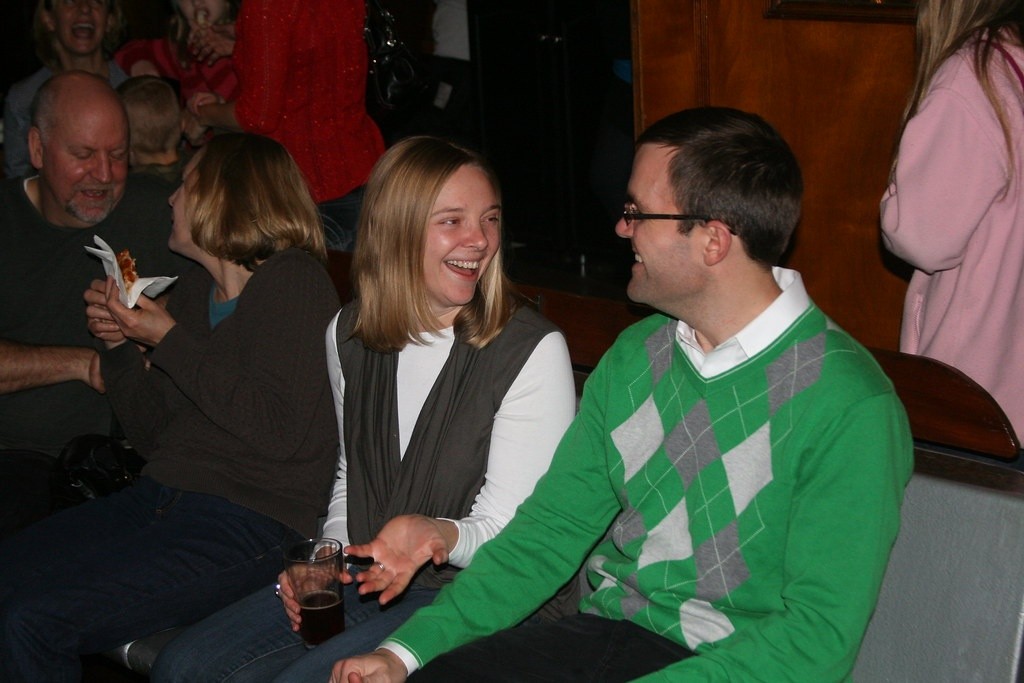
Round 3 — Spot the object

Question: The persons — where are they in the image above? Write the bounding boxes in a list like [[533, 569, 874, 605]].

[[0, 0, 235, 177], [328, 106, 913, 683], [180, 0, 384, 254], [149, 137, 581, 683], [0, 133, 342, 683], [0, 69, 194, 544], [879, 0, 1024, 447]]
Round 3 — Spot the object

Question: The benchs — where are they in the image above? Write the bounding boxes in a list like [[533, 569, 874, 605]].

[[48, 361, 1024, 683]]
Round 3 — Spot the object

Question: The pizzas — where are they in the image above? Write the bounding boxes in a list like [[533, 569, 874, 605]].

[[117, 248, 139, 287]]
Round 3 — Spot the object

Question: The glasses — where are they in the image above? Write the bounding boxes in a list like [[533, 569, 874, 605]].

[[623, 201, 738, 235]]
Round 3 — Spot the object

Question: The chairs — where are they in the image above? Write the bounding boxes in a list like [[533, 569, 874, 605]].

[[515, 283, 644, 372], [867, 347, 1024, 470]]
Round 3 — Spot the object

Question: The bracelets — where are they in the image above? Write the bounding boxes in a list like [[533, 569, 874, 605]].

[[215, 94, 220, 104]]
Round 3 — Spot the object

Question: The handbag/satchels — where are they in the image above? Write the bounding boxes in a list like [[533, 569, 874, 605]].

[[40, 433, 147, 509]]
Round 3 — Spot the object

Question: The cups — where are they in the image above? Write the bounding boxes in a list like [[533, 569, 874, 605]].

[[284, 537, 346, 651]]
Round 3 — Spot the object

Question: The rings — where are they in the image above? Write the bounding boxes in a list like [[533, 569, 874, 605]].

[[276, 584, 281, 596], [372, 562, 384, 570]]
